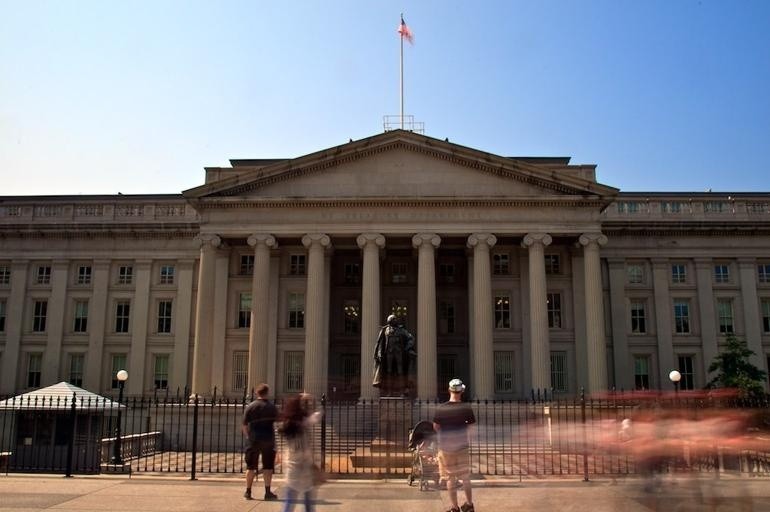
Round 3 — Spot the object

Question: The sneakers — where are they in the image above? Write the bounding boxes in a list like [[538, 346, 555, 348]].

[[445, 506, 460, 512], [264, 492, 278, 501], [243, 490, 252, 498], [461, 502, 474, 512]]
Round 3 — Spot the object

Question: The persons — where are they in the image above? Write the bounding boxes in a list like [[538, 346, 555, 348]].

[[370, 315, 416, 393], [432, 378, 475, 511], [240, 384, 284, 499], [276, 391, 324, 511]]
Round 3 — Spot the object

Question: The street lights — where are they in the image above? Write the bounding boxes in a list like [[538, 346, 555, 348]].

[[667, 369, 689, 468], [108, 369, 129, 464]]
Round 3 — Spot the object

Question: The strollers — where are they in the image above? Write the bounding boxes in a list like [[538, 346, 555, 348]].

[[408, 420, 447, 492]]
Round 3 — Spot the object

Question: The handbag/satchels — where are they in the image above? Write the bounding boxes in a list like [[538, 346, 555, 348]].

[[311, 463, 327, 488]]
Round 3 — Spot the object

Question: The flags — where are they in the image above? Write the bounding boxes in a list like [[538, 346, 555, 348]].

[[398, 17, 413, 39]]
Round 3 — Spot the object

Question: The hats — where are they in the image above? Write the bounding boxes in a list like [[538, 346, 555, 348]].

[[448, 379, 466, 392]]
